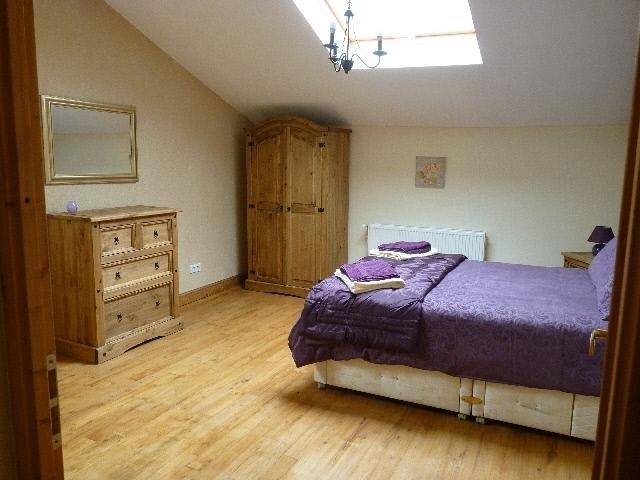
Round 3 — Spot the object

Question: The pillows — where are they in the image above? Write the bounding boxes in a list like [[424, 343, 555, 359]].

[[588, 234, 618, 321]]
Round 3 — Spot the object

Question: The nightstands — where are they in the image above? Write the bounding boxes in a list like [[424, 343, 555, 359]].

[[560, 251, 596, 269]]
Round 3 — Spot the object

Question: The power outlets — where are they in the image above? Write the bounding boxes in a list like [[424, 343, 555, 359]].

[[189, 261, 202, 274]]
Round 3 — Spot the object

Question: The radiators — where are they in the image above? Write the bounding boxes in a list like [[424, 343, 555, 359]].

[[361, 223, 487, 261]]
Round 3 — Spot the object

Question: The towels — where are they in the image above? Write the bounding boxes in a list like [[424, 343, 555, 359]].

[[334, 240, 441, 295]]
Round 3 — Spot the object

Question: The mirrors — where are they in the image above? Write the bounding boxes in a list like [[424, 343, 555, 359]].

[[39, 90, 140, 186]]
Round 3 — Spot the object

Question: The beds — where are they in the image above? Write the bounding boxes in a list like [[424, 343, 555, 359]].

[[287, 235, 617, 445]]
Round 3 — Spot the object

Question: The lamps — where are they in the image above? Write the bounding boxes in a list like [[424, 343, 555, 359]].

[[321, 0, 386, 78], [587, 225, 617, 256]]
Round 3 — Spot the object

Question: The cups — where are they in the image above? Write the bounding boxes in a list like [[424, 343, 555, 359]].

[[67, 201, 79, 215]]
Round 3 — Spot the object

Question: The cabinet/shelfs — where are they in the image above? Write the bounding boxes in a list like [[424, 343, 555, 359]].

[[46, 203, 186, 365], [241, 113, 353, 303]]
